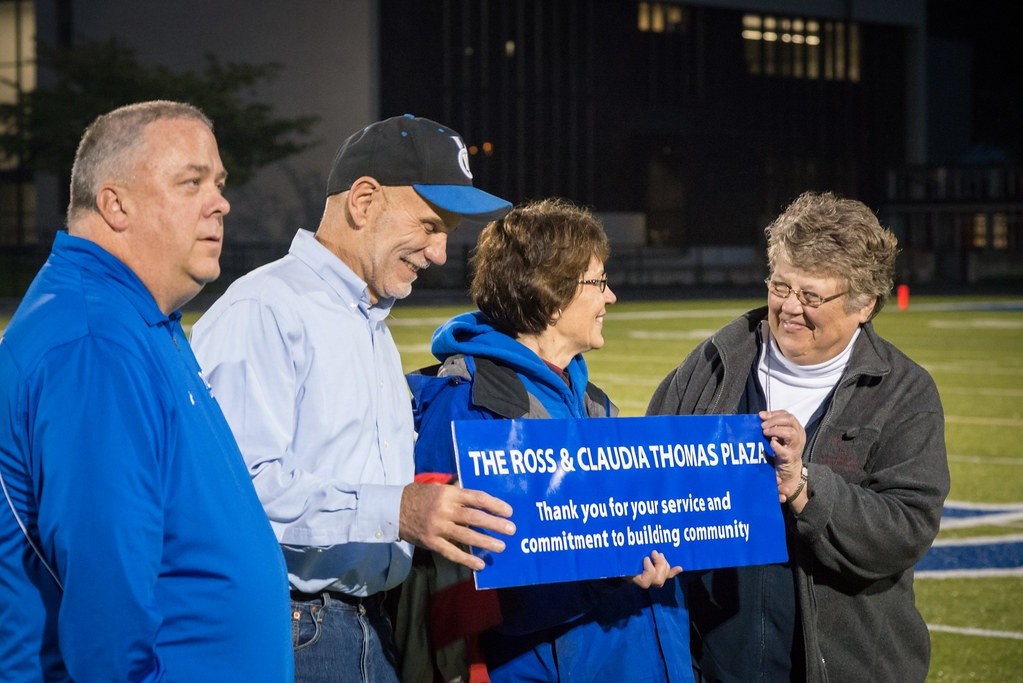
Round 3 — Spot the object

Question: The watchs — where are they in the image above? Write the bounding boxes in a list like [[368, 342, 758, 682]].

[[787, 466, 808, 502]]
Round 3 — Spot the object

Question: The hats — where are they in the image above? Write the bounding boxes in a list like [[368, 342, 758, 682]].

[[326, 113, 513, 219]]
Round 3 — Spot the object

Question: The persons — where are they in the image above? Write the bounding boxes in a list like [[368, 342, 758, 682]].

[[407, 205, 690, 683], [645, 190, 951, 683], [189, 114, 516, 683], [0, 100, 295, 683]]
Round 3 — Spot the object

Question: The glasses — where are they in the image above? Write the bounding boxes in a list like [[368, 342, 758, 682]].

[[577, 273, 614, 291], [764, 274, 849, 307]]
